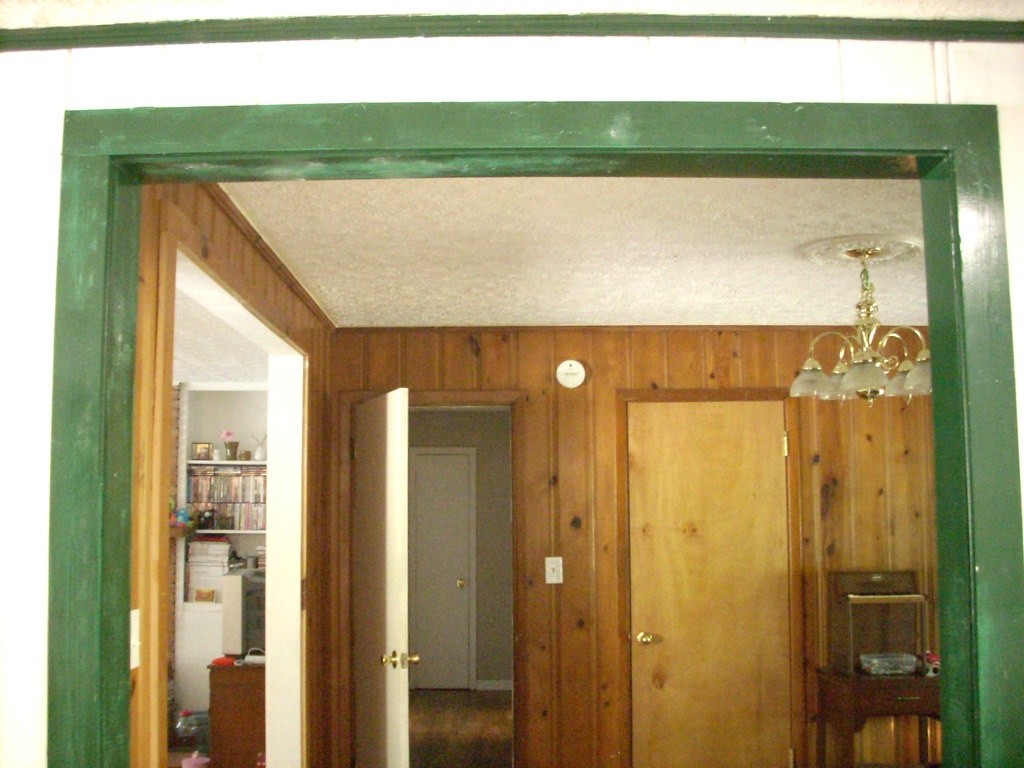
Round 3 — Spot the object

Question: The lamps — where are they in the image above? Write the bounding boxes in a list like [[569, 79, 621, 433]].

[[790, 233, 932, 410]]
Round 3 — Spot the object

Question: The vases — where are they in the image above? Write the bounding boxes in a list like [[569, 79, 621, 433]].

[[224, 441, 239, 462], [255, 446, 267, 461]]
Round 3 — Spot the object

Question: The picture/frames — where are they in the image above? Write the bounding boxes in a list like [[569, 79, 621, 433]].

[[190, 442, 214, 460], [194, 587, 216, 604]]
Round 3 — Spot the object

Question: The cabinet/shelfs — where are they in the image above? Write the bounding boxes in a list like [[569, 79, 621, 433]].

[[205, 657, 266, 768], [185, 459, 267, 535]]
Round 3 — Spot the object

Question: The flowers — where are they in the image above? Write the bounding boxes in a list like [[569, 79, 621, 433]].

[[220, 430, 235, 442]]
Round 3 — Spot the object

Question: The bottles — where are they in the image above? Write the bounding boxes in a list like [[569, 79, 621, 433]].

[[176, 710, 199, 753]]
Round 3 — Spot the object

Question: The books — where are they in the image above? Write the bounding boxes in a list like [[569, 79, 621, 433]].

[[187, 466, 266, 531]]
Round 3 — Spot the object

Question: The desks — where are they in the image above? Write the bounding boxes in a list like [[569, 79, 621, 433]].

[[814, 664, 941, 768]]
